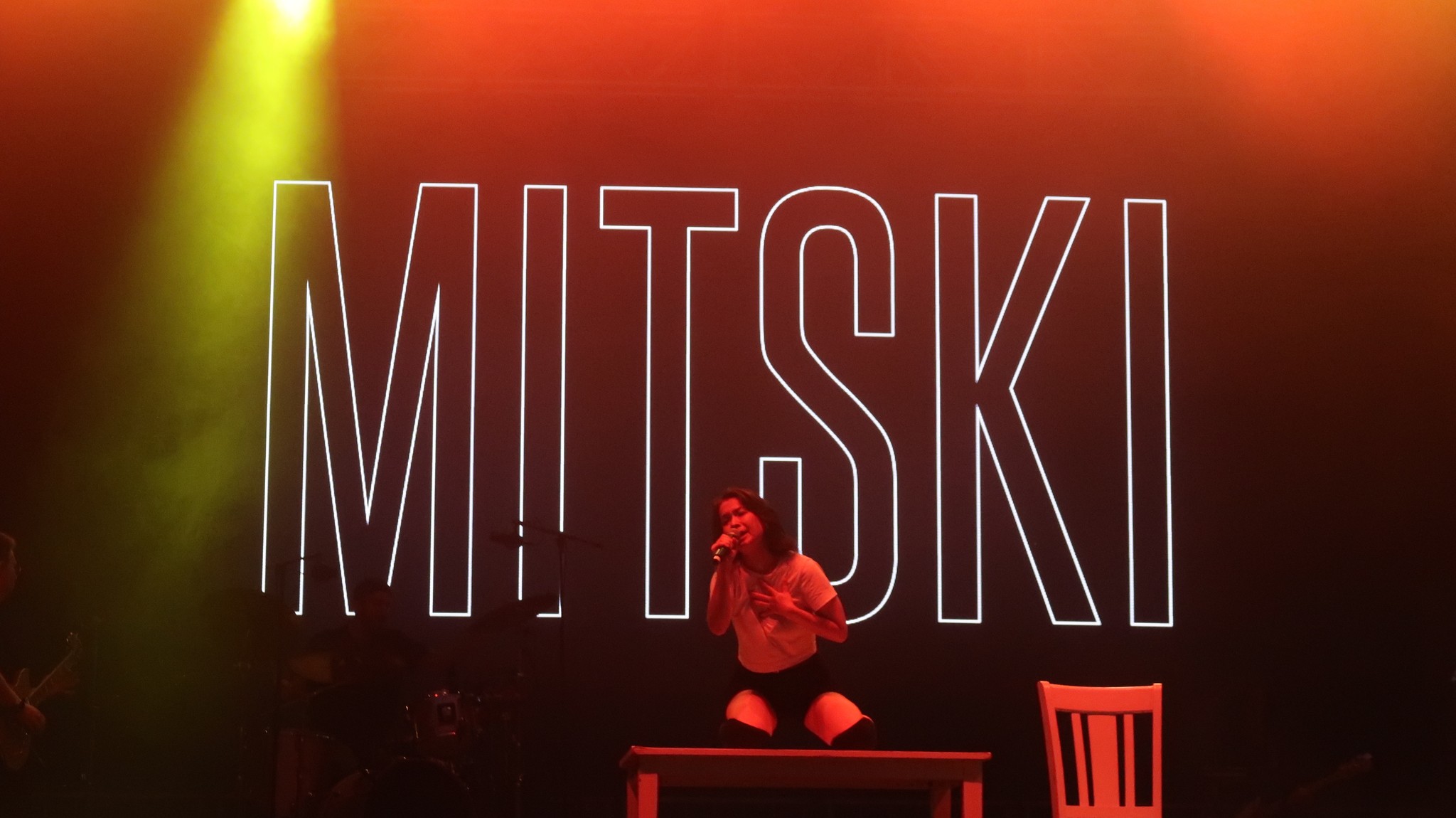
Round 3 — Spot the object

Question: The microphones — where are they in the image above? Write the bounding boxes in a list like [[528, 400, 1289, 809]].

[[713, 529, 739, 563]]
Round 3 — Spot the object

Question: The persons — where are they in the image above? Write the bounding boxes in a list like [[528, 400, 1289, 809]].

[[0, 533, 48, 818], [302, 578, 423, 769], [707, 487, 877, 750]]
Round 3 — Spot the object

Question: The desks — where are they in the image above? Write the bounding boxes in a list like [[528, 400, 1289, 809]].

[[617, 745, 993, 818]]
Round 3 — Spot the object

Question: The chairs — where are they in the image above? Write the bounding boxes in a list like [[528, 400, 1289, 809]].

[[1035, 680, 1162, 818]]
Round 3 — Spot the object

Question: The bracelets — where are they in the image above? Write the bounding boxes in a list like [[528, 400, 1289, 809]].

[[8, 697, 25, 715]]
